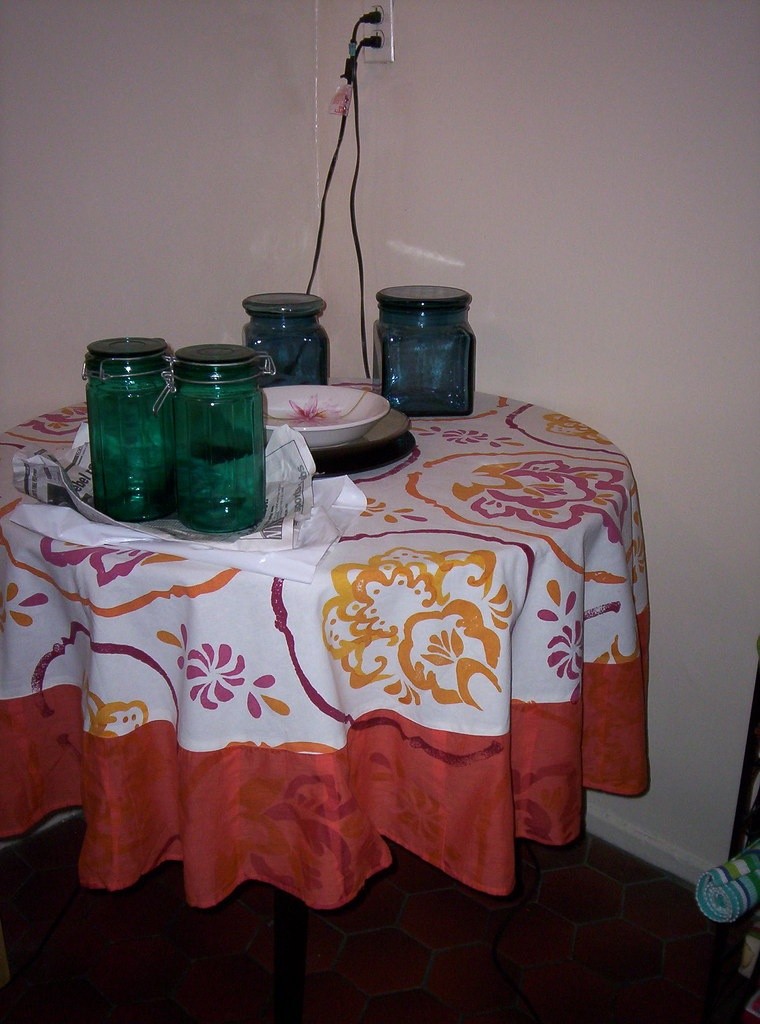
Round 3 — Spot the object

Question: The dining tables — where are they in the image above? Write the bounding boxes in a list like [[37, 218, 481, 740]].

[[0, 375, 651, 1024]]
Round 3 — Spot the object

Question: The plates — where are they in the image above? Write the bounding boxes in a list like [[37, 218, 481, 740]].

[[262, 384, 391, 444], [309, 410, 412, 461], [313, 431, 416, 479]]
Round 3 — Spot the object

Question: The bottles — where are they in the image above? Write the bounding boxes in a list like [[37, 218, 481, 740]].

[[169, 343, 269, 535], [241, 293, 329, 387], [372, 286, 474, 418], [82, 338, 173, 522]]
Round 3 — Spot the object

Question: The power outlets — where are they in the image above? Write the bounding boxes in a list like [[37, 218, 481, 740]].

[[362, 1, 395, 64]]
